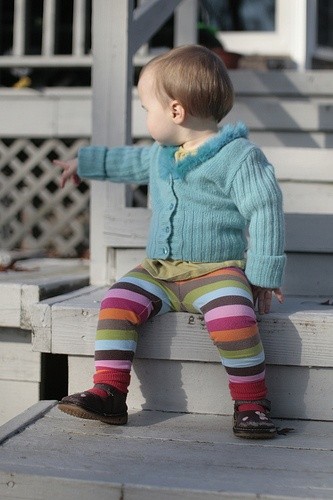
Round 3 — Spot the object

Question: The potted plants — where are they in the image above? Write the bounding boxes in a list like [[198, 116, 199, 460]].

[[198, 26, 244, 70]]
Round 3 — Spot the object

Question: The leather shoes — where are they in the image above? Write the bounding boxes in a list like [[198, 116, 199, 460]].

[[56, 383, 130, 424], [233, 397, 276, 436]]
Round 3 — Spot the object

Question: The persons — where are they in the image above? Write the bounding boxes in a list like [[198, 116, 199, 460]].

[[52, 45, 288, 438]]
